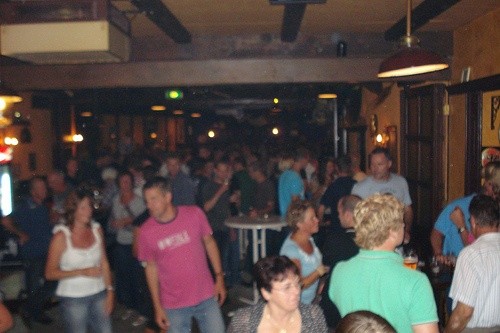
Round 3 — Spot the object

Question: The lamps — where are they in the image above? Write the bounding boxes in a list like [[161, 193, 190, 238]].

[[377, 0, 449, 78]]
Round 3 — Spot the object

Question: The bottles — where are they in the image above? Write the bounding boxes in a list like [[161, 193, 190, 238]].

[[4, 239, 18, 257]]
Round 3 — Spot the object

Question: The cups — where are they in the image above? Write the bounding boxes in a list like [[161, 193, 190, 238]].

[[403, 256, 418, 270], [230, 202, 238, 216]]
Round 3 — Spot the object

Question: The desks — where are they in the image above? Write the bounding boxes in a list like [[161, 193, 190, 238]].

[[223, 215, 288, 307]]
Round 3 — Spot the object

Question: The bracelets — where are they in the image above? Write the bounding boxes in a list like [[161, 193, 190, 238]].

[[106, 287, 113, 292], [215, 272, 223, 276], [457, 226, 465, 233]]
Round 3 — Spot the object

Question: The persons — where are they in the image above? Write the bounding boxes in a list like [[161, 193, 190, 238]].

[[225, 255, 329, 333], [137, 177, 228, 333], [45, 189, 115, 333], [276, 146, 500, 333], [1, 143, 298, 333]]
